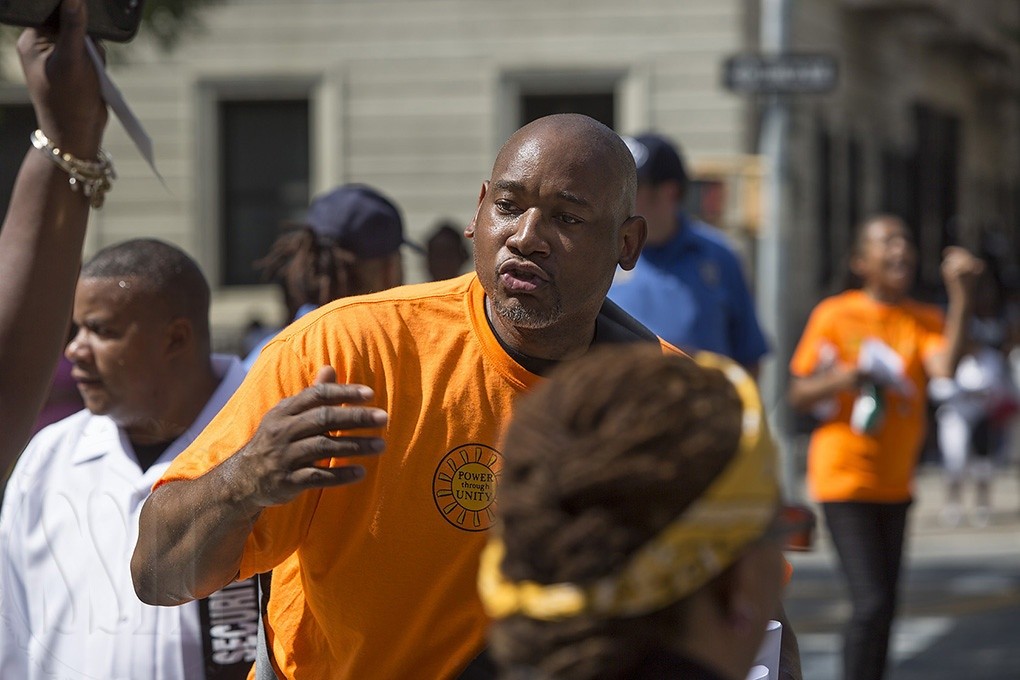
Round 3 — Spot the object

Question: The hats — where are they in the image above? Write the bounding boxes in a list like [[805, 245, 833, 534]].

[[623, 137, 681, 185], [295, 186, 403, 256]]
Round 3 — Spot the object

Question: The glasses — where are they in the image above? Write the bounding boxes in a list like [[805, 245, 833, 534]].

[[779, 507, 814, 551]]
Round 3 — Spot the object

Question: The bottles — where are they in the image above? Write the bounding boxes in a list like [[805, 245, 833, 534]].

[[850, 358, 885, 433]]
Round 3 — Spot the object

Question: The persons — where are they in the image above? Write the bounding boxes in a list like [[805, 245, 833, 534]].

[[427, 225, 472, 282], [0, 239, 260, 680], [785, 213, 982, 680], [0, 0, 109, 494], [245, 186, 406, 374], [130, 112, 696, 680], [603, 136, 770, 376], [477, 343, 785, 680], [25, 326, 86, 438]]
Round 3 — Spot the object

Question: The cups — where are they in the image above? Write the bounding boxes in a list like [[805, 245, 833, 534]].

[[747, 620, 782, 680]]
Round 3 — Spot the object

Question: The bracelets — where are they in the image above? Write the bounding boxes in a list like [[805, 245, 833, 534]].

[[29, 130, 116, 209]]
